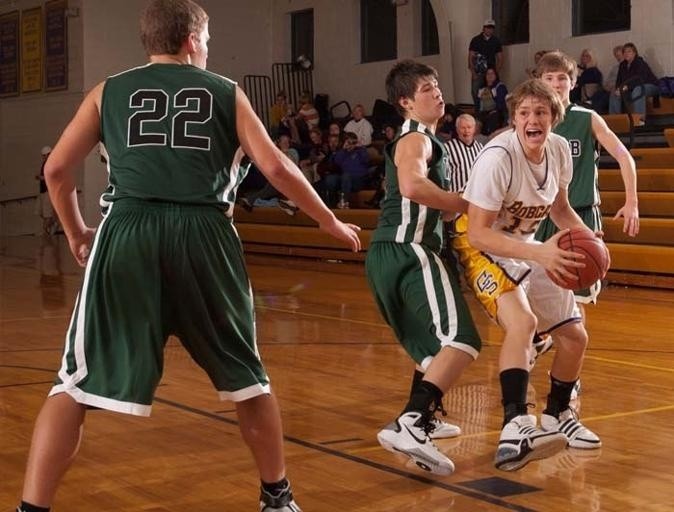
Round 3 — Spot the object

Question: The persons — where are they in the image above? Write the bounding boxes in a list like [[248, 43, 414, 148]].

[[366, 62, 482, 476], [17, 2, 361, 511], [466, 20, 659, 136], [453, 79, 604, 473], [237, 88, 407, 216], [440, 114, 484, 292], [34, 146, 54, 238], [529, 51, 640, 400]]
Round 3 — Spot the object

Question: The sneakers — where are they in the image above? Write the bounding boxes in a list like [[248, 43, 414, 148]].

[[254, 489, 304, 512], [35, 222, 59, 239], [376, 409, 456, 477], [275, 198, 297, 217], [541, 404, 604, 451], [424, 413, 463, 439], [237, 196, 253, 213], [493, 413, 569, 473]]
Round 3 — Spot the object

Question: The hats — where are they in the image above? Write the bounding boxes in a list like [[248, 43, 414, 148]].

[[483, 19, 497, 28]]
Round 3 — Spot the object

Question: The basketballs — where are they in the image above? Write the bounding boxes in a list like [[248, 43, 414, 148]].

[[546, 226, 609, 290]]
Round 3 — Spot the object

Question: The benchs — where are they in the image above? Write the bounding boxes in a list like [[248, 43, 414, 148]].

[[236, 98, 674, 293]]
